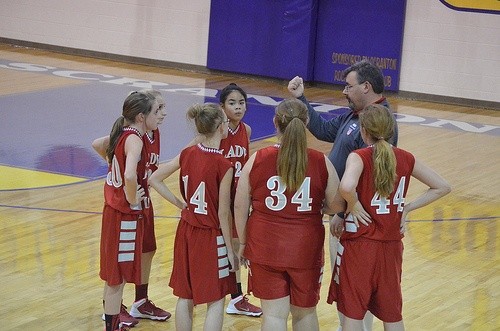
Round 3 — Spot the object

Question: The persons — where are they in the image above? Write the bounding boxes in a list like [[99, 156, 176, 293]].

[[90, 89, 172, 322], [233, 98, 346, 331], [98, 90, 161, 331], [147, 102, 238, 331], [287, 59, 397, 331], [326, 104, 452, 331], [181, 83, 263, 317]]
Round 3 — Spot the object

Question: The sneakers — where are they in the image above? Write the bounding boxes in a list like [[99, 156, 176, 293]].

[[225, 293, 263, 316], [130, 296, 171, 320], [102, 304, 139, 327]]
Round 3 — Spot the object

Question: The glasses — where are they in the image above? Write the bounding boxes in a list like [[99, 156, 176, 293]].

[[344, 81, 367, 92]]
